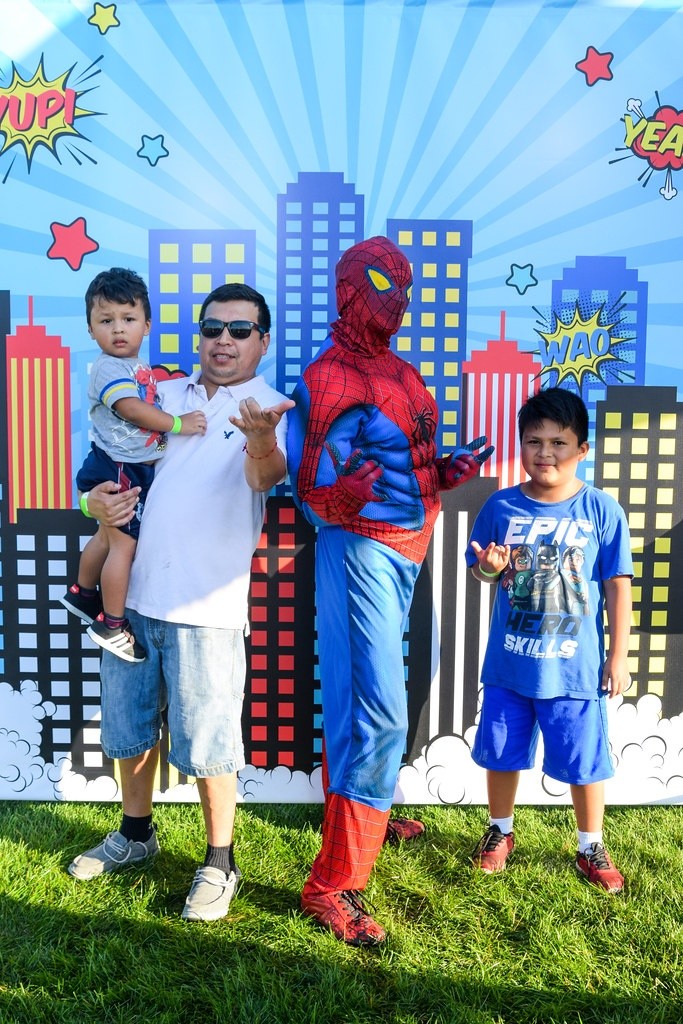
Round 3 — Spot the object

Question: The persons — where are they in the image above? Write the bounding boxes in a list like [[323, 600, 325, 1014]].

[[72, 288, 296, 922], [298, 236, 495, 945], [58, 267, 207, 663], [464, 387, 634, 893]]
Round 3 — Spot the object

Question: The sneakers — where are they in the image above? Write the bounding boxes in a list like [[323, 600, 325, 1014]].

[[302, 889, 386, 945], [86, 612, 146, 662], [180, 863, 243, 922], [59, 584, 102, 625], [575, 842, 624, 892], [468, 824, 514, 875], [68, 821, 160, 880], [383, 817, 424, 843]]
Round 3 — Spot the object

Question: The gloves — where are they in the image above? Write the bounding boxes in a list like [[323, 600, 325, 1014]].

[[323, 439, 390, 503], [443, 436, 495, 490]]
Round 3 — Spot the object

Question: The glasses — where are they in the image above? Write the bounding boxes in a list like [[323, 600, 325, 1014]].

[[199, 319, 265, 339]]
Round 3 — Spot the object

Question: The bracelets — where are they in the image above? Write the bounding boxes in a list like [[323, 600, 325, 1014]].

[[169, 414, 182, 434], [80, 492, 93, 518], [242, 441, 277, 460], [479, 563, 500, 577]]
